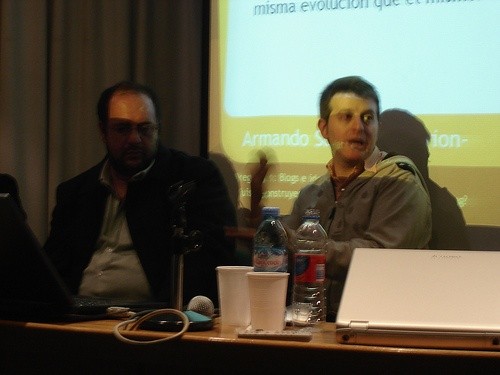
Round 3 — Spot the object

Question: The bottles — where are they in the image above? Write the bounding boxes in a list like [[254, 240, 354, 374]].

[[252, 206, 289, 275], [292, 209, 329, 332]]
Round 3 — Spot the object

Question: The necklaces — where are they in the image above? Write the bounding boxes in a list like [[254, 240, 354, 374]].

[[99, 194, 125, 276]]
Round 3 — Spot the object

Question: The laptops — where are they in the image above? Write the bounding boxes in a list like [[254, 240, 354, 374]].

[[336, 247, 500, 351], [0, 192, 172, 319]]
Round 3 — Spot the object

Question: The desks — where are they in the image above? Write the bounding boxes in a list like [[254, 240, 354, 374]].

[[0, 318, 500, 375]]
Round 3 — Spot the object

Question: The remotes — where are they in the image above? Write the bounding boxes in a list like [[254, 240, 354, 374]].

[[237, 327, 312, 342], [189, 296, 221, 318]]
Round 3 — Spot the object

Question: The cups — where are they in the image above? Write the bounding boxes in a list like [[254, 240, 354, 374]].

[[215, 266, 255, 327], [246, 271, 290, 332]]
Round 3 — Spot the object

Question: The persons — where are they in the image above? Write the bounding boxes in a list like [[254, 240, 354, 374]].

[[0, 80, 237, 315], [279, 76, 431, 323]]
